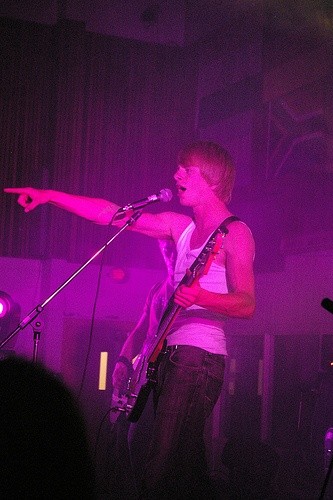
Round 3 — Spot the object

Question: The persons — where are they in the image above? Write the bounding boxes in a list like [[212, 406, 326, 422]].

[[0, 356, 93, 500], [5, 141, 255, 500], [112, 237, 178, 500]]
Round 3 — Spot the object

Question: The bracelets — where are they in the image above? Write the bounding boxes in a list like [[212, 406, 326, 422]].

[[115, 356, 131, 366]]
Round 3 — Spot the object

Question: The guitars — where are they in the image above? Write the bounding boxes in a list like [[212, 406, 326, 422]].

[[108, 227, 225, 424]]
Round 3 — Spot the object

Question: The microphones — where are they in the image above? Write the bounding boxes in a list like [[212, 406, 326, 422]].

[[321, 297, 333, 314], [118, 189, 173, 213]]
